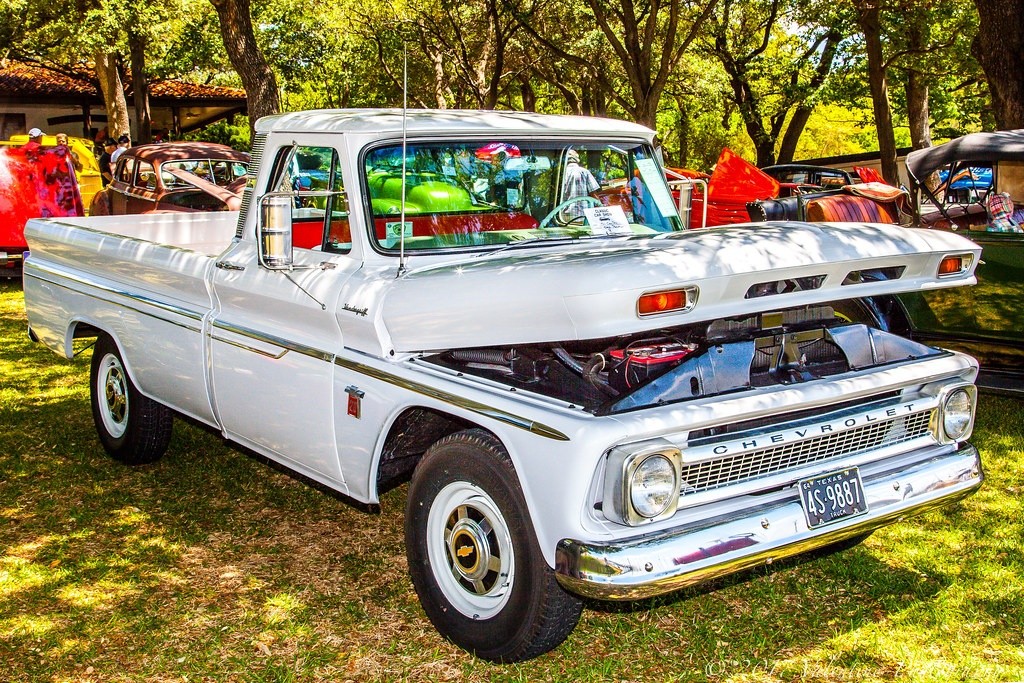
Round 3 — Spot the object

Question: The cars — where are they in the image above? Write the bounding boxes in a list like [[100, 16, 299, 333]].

[[86, 140, 251, 216], [591, 129, 1023, 403]]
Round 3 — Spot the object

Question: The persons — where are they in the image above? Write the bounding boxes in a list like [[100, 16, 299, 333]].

[[21, 127, 42, 148], [561, 149, 602, 224], [160, 128, 169, 142], [56, 133, 83, 173], [99, 134, 130, 189], [488, 151, 522, 208], [94, 126, 109, 142]]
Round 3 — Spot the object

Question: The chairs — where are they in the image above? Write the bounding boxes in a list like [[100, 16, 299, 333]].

[[123, 174, 157, 188]]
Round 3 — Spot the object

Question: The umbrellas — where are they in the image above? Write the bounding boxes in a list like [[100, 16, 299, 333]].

[[475, 142, 520, 162]]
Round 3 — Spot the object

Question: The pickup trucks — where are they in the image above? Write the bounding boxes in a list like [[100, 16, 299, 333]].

[[19, 105, 985, 672]]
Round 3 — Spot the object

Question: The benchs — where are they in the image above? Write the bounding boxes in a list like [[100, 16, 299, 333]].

[[933, 211, 992, 231], [292, 214, 540, 249], [805, 194, 898, 224]]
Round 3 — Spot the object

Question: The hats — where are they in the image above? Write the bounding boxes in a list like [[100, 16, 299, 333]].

[[118, 136, 130, 143], [29, 128, 46, 138], [106, 138, 118, 145]]
